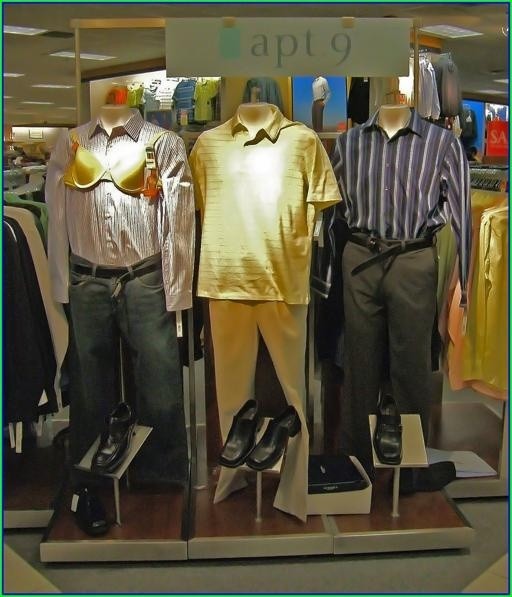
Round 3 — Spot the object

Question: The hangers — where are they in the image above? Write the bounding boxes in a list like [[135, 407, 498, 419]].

[[468, 164, 509, 192], [8, 170, 19, 198], [127, 73, 213, 92], [441, 52, 454, 67], [408, 47, 430, 65]]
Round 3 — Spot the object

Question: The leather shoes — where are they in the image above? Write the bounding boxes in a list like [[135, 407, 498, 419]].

[[92, 403, 135, 474], [393, 462, 457, 494], [218, 399, 263, 469], [75, 488, 108, 533], [246, 405, 300, 470], [374, 392, 403, 465]]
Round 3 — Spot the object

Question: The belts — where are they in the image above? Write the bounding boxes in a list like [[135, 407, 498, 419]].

[[348, 235, 432, 277], [73, 263, 163, 300]]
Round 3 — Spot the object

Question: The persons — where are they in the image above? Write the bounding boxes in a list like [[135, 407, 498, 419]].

[[323, 101, 472, 454], [311, 75, 329, 133], [185, 101, 342, 523], [45, 102, 199, 486]]
